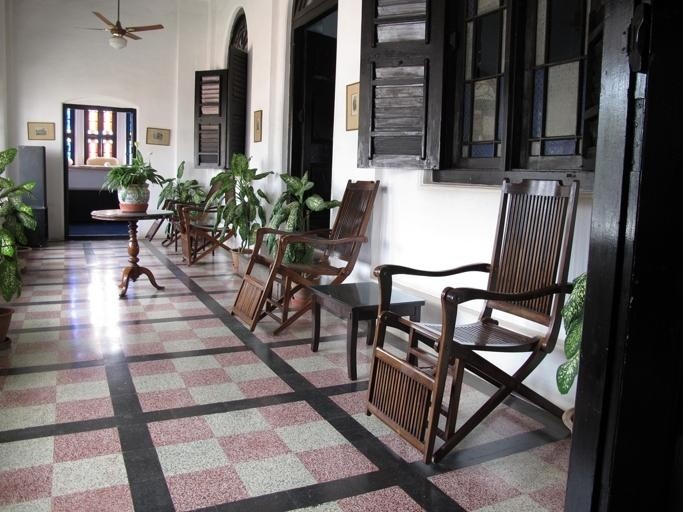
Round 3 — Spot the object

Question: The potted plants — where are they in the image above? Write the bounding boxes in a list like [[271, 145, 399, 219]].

[[0, 148, 40, 351], [100, 142, 165, 212], [266, 170, 342, 314], [555, 272, 586, 439], [202, 152, 275, 275]]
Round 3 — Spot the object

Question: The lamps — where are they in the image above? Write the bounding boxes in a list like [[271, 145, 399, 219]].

[[108, 30, 127, 49]]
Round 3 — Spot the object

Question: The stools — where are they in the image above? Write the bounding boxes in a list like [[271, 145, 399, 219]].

[[307, 282, 426, 380]]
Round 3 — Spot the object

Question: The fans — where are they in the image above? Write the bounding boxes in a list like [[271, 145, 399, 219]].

[[80, 0, 164, 40]]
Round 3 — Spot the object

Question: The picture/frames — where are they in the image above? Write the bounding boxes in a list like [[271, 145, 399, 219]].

[[146, 128, 171, 146], [346, 81, 360, 130], [253, 110, 262, 142], [27, 121, 56, 140]]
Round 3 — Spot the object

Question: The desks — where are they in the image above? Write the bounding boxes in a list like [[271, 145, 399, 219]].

[[90, 208, 174, 297]]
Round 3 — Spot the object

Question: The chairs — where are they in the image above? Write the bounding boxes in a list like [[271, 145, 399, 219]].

[[365, 176, 580, 464], [228, 180, 380, 335], [145, 187, 199, 247], [176, 180, 236, 265]]
